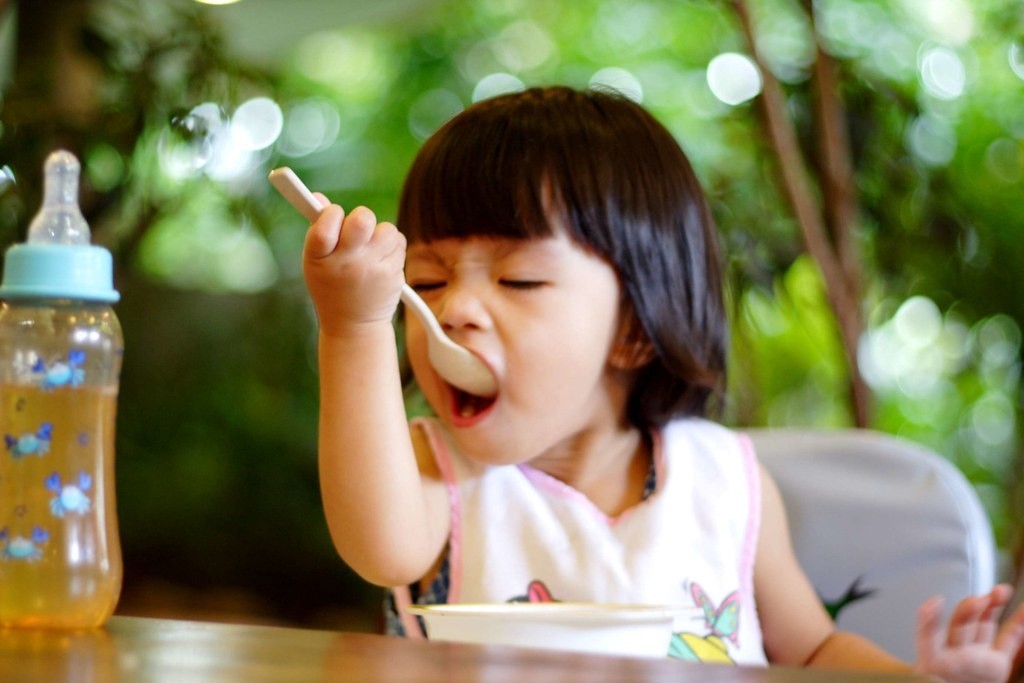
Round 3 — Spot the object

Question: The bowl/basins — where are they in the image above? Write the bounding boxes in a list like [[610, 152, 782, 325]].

[[404, 600, 707, 659]]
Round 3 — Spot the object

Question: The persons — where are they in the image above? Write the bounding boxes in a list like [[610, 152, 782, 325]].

[[299, 84, 1024, 683]]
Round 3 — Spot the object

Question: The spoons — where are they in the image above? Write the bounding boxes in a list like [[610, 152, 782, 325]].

[[266, 166, 497, 396]]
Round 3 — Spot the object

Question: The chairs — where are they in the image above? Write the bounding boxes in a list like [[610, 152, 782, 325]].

[[730, 414, 1009, 669]]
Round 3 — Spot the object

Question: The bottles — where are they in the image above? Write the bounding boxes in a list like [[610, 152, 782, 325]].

[[0, 148, 127, 631]]
